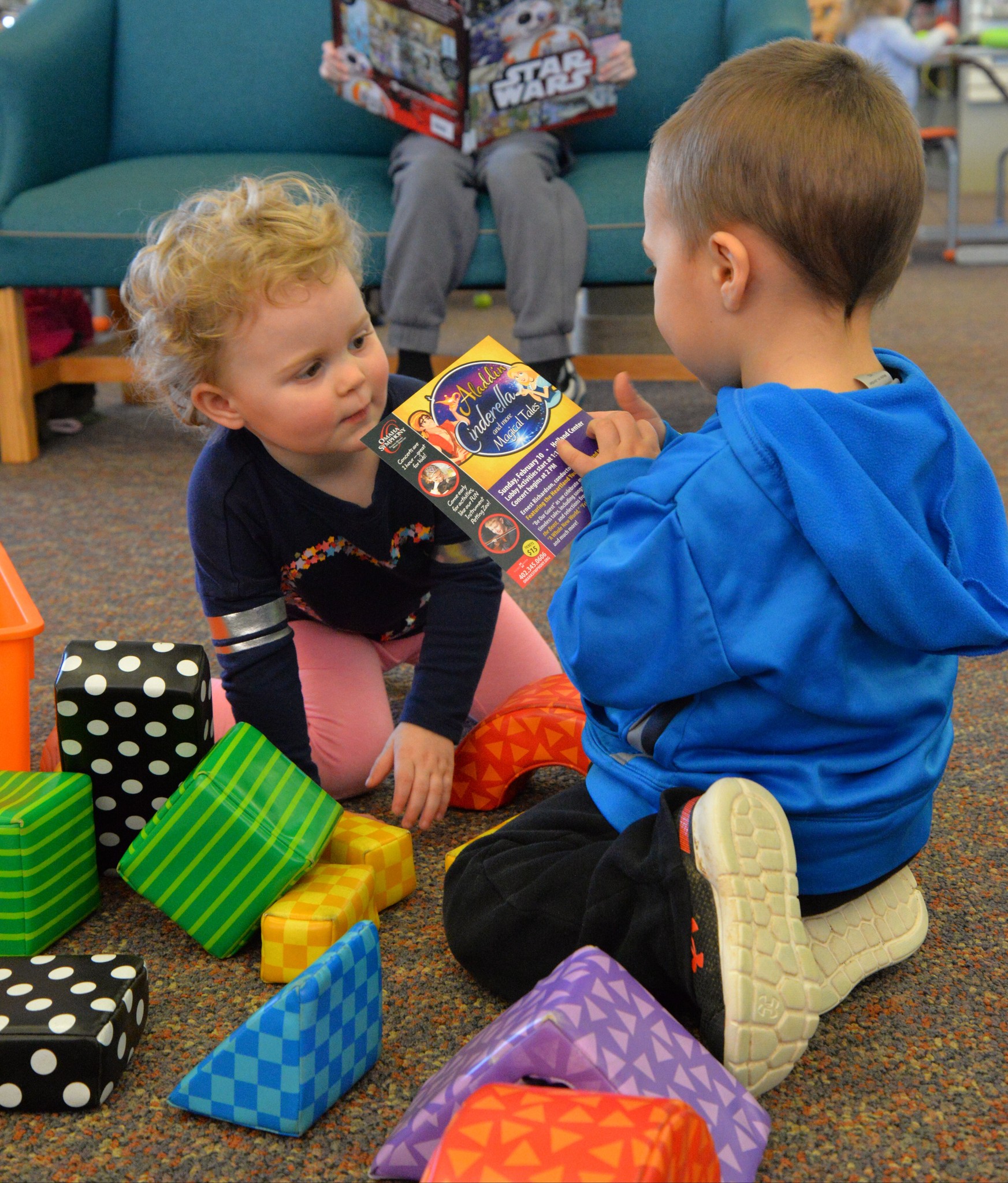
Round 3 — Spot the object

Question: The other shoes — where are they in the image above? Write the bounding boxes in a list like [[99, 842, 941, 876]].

[[673, 776, 821, 1100], [801, 865, 928, 1015]]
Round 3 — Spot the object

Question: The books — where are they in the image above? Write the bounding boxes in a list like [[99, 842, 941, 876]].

[[328, 0, 618, 154]]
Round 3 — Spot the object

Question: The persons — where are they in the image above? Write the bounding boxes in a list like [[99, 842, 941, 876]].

[[320, 0, 635, 401], [124, 171, 581, 799], [835, 0, 958, 130], [435, 38, 1008, 1100]]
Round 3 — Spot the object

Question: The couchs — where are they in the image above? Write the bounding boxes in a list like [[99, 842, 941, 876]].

[[0, 0, 816, 464]]
